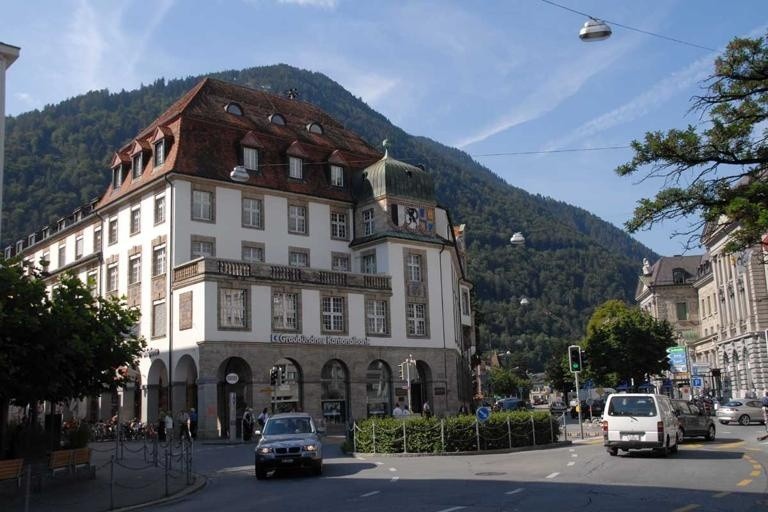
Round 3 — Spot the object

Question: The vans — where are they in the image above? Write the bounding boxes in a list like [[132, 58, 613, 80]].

[[601, 393, 678, 456]]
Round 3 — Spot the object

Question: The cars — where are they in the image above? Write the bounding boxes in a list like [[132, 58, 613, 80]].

[[716, 399, 762, 425], [670, 399, 715, 444], [255, 412, 325, 480], [551, 401, 566, 414]]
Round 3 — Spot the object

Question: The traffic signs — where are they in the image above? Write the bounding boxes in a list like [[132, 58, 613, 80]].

[[665, 346, 711, 387]]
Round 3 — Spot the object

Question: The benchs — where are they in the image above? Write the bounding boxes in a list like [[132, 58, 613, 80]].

[[46, 447, 92, 477], [0, 458, 25, 488]]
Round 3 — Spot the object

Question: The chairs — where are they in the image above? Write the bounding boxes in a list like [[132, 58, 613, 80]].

[[271, 423, 287, 434]]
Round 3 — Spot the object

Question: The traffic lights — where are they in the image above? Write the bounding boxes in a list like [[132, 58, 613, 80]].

[[268, 368, 285, 385], [567, 345, 582, 373], [397, 360, 420, 383]]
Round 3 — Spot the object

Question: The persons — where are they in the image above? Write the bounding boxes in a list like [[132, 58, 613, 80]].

[[244, 406, 253, 440], [392, 401, 403, 417], [95, 407, 197, 446], [257, 407, 268, 433], [761, 391, 768, 432], [422, 399, 431, 418], [402, 404, 411, 415]]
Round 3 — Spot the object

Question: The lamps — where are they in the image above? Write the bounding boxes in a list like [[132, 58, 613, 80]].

[[579, 19, 613, 43], [519, 298, 530, 306], [510, 231, 526, 245], [229, 165, 250, 183]]
